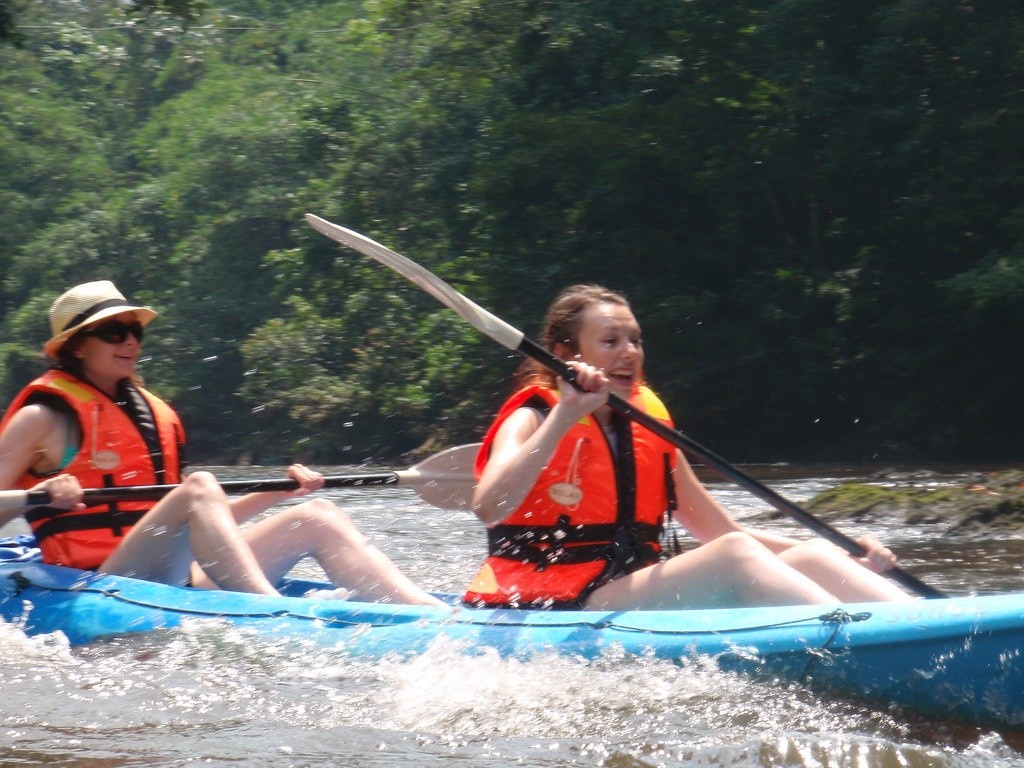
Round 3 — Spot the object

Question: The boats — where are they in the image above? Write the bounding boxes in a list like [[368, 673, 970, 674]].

[[1, 535, 1024, 726]]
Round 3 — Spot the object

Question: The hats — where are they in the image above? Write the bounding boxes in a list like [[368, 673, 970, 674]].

[[42, 281, 157, 357]]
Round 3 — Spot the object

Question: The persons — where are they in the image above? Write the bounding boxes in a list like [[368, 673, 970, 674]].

[[458, 286, 908, 611], [0, 282, 446, 606]]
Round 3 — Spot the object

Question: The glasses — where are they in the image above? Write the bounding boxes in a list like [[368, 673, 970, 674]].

[[75, 318, 144, 345]]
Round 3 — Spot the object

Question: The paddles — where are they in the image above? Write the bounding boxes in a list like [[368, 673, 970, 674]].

[[306, 211, 953, 599], [0, 442, 484, 511]]
